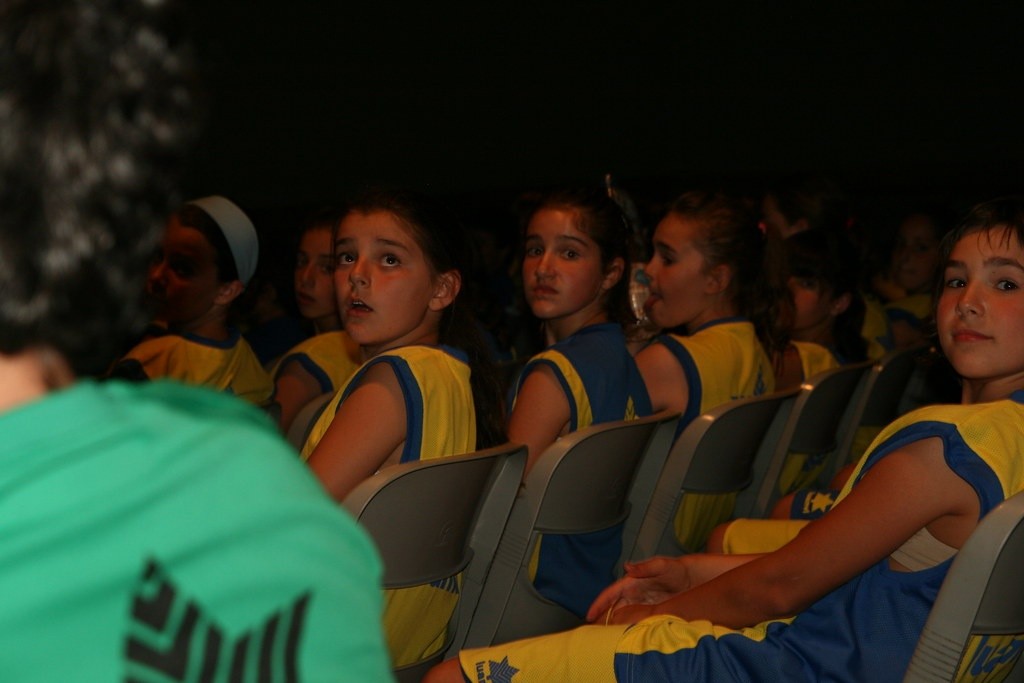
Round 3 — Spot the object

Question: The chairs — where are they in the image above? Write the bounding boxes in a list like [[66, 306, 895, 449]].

[[634, 385, 805, 570], [826, 335, 931, 479], [468, 404, 686, 653], [759, 356, 884, 522], [906, 493, 1024, 683], [340, 441, 529, 683]]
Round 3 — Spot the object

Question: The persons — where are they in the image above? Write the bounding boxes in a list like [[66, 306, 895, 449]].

[[420, 199, 1024, 683], [110, 180, 948, 668], [0, 0, 396, 683]]
